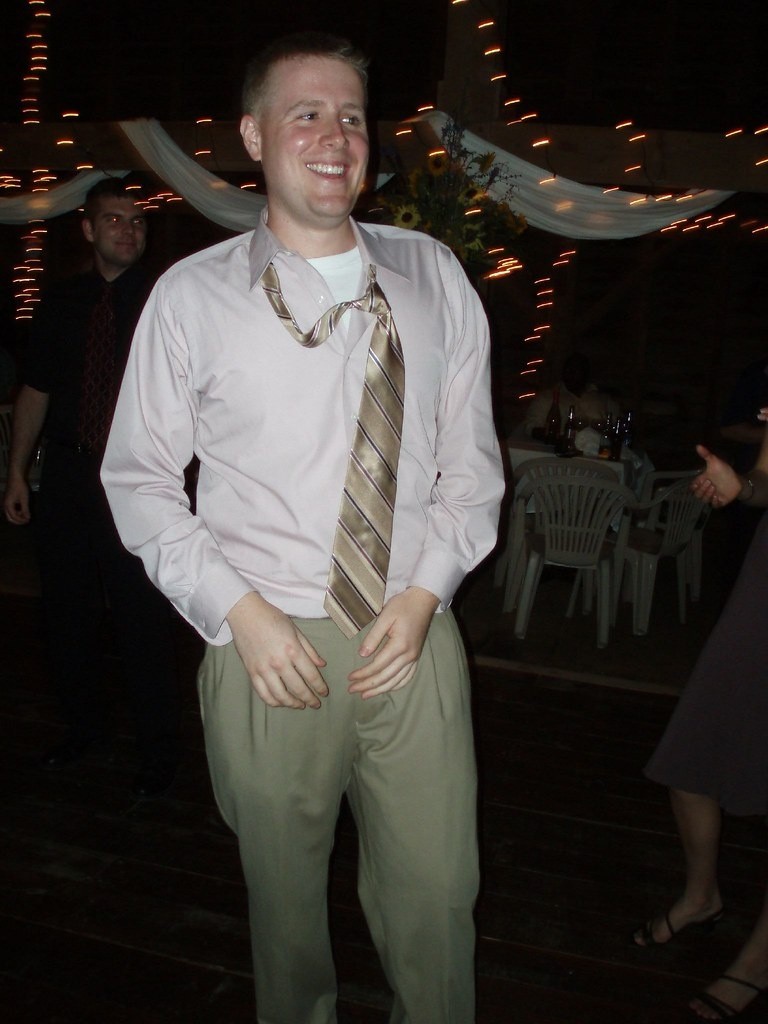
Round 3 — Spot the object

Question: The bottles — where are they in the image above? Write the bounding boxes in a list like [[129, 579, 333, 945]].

[[559, 404, 578, 458], [589, 407, 636, 461], [543, 385, 562, 446]]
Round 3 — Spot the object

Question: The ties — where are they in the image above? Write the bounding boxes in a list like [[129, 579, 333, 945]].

[[75, 283, 120, 451], [264, 261, 405, 639]]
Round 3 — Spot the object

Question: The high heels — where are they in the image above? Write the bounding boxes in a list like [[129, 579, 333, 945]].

[[684, 974, 763, 1024], [628, 908, 723, 947]]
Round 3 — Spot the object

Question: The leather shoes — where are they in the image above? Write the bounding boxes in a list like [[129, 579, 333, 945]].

[[41, 726, 118, 772], [128, 745, 179, 800]]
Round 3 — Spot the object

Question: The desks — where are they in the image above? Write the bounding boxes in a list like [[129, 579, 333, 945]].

[[498, 434, 654, 580]]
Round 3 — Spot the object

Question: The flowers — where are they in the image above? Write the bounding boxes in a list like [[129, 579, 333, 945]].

[[363, 83, 532, 272]]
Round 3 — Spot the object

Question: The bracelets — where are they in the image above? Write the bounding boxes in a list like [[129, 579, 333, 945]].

[[741, 474, 754, 502]]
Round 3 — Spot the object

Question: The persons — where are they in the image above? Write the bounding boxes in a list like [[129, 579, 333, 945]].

[[100, 23, 507, 1024], [636, 405, 768, 1023], [2, 176, 185, 801], [524, 350, 621, 433], [718, 357, 768, 559]]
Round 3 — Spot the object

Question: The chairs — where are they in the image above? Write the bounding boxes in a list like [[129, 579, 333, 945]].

[[0, 405, 48, 491], [493, 455, 735, 649]]
